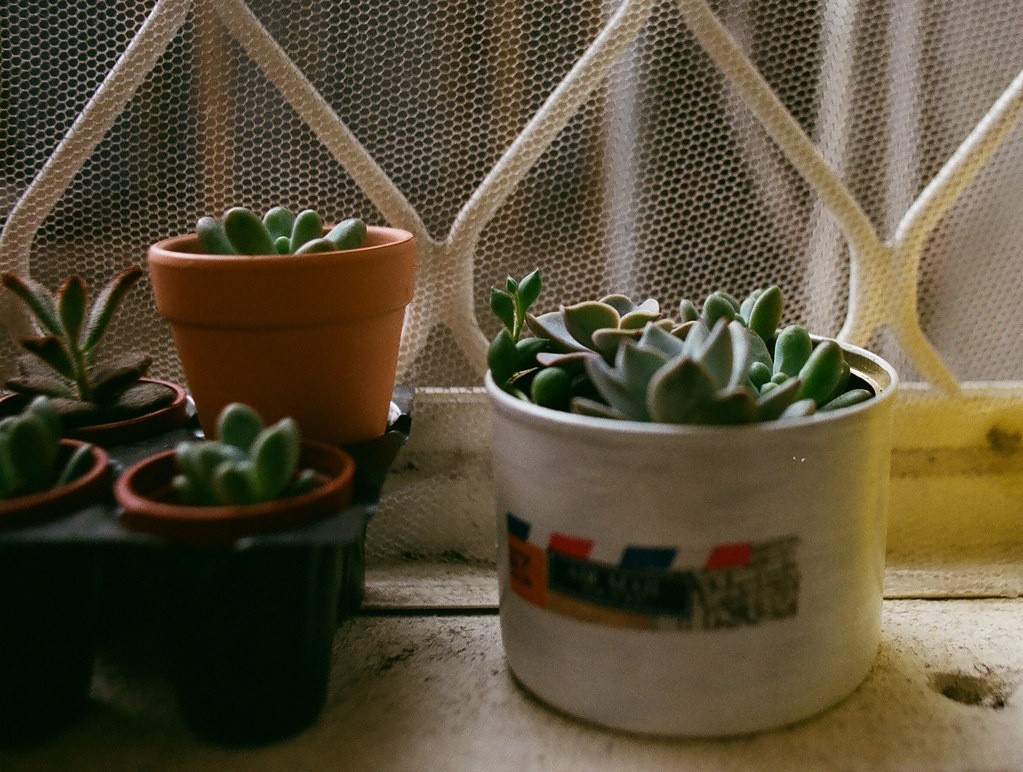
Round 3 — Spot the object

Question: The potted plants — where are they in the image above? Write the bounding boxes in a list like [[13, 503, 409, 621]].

[[482, 270, 898, 736], [0, 268, 185, 457], [148, 206, 414, 441], [0, 394, 109, 743], [112, 406, 355, 741]]
